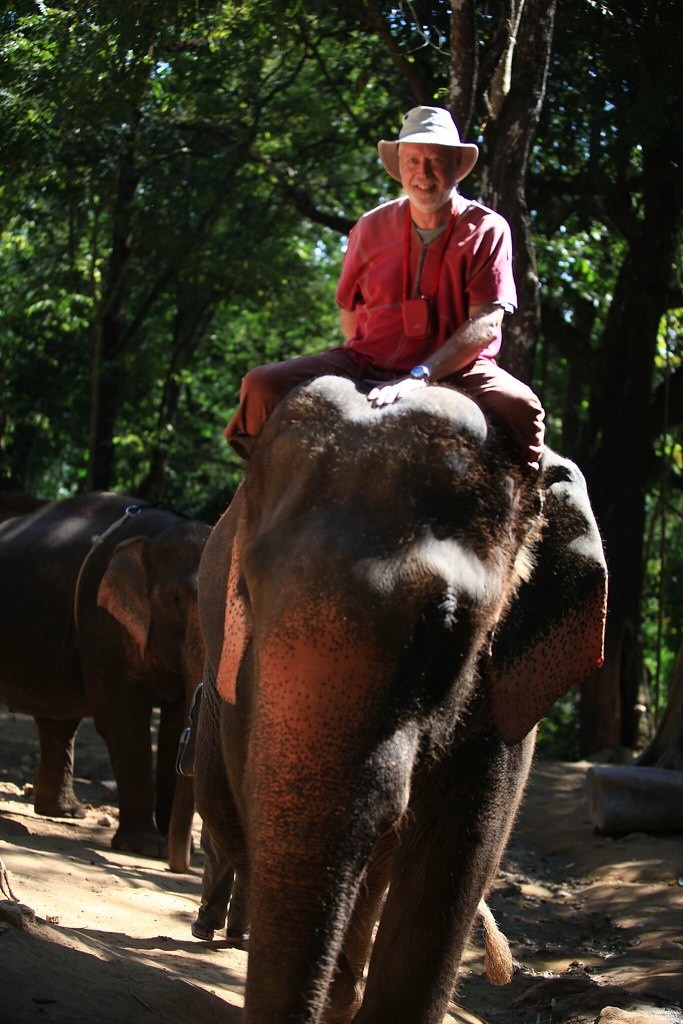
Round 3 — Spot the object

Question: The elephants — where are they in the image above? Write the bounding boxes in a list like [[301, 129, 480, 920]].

[[192, 364, 612, 1022], [0, 493, 216, 872]]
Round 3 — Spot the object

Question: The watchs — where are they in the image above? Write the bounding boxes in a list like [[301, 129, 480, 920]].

[[410, 364, 432, 382]]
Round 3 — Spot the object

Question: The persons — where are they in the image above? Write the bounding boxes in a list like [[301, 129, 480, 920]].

[[221, 106, 545, 470]]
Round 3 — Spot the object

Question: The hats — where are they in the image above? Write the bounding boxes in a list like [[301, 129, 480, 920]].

[[378, 106, 479, 187]]
